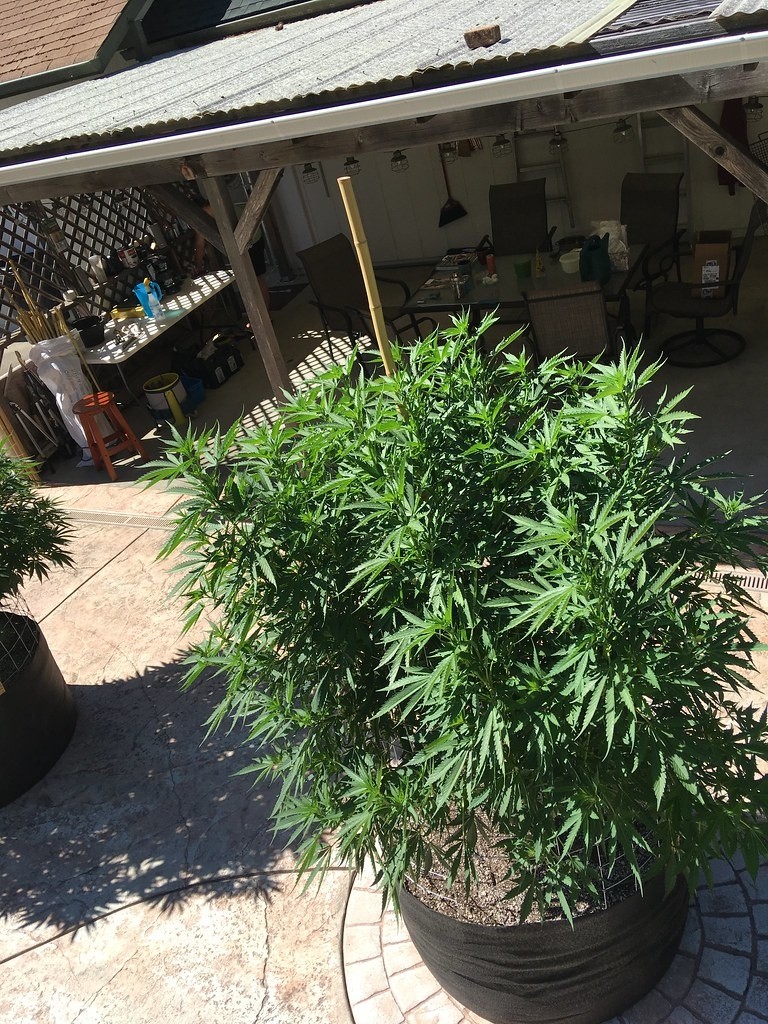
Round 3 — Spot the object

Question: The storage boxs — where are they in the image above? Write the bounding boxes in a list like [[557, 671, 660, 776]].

[[690, 230, 733, 302]]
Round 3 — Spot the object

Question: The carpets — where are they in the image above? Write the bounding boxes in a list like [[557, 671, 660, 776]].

[[234, 283, 309, 312]]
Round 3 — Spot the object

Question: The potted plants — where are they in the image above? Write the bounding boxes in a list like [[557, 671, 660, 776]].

[[0, 436, 77, 807], [136, 301, 768, 1024]]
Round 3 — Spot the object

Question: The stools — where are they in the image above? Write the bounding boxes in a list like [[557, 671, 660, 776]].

[[72, 392, 147, 481]]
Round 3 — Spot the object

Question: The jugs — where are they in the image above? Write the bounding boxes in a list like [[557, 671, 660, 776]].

[[132, 281, 162, 319]]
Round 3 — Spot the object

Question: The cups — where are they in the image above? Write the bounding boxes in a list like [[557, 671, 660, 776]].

[[513, 255, 532, 278], [487, 254, 495, 275], [559, 252, 579, 274]]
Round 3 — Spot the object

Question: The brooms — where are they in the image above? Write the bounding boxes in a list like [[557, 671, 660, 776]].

[[438, 145, 469, 229]]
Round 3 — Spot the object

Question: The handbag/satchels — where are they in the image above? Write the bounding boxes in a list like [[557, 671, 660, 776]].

[[555, 235, 586, 251], [578, 232, 612, 282]]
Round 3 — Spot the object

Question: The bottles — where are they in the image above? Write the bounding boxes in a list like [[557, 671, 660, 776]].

[[147, 292, 166, 324], [451, 272, 465, 301]]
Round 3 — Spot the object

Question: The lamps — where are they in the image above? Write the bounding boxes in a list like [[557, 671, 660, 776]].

[[302, 162, 320, 185], [740, 97, 763, 123], [344, 156, 361, 178], [548, 132, 570, 155], [613, 119, 635, 145], [391, 151, 410, 173], [492, 134, 512, 158], [437, 142, 458, 167]]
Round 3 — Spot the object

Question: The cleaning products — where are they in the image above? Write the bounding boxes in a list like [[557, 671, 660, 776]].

[[143, 276, 166, 324]]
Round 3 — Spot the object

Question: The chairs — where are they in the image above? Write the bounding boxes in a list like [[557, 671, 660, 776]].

[[311, 300, 449, 388], [620, 170, 687, 339], [474, 177, 557, 255], [296, 234, 413, 378], [649, 199, 768, 369]]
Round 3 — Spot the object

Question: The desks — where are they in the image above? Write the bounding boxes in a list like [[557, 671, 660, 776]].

[[399, 243, 647, 386], [78, 270, 238, 431]]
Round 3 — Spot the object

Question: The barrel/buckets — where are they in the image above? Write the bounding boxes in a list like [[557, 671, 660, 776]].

[[143, 373, 190, 428], [71, 315, 104, 348]]
